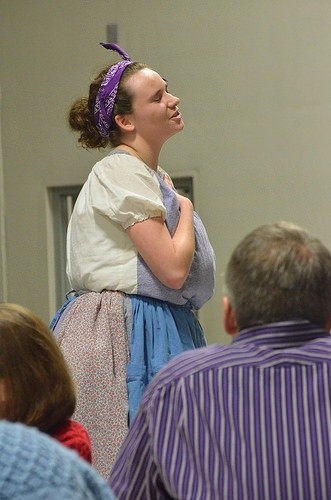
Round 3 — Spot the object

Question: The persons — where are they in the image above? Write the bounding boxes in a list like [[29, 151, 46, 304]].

[[49, 43, 216, 486], [105, 222, 331, 500], [0, 300, 93, 466]]
